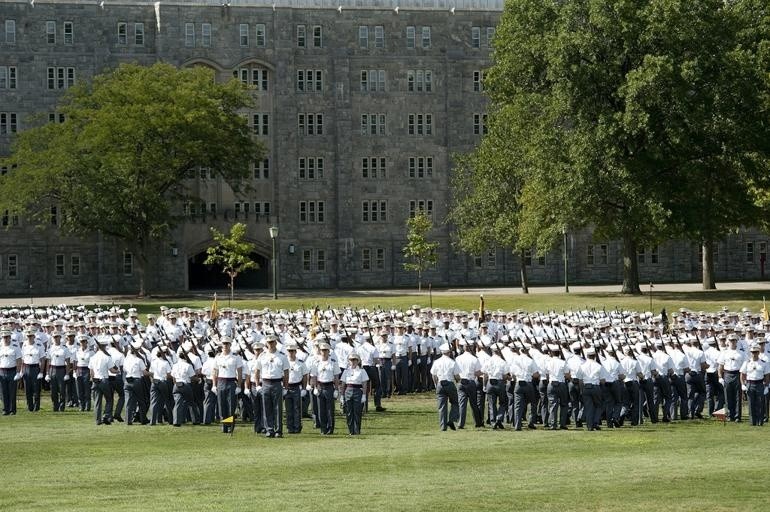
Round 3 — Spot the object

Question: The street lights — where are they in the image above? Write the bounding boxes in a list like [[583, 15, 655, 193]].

[[267, 222, 286, 300], [557, 220, 581, 295]]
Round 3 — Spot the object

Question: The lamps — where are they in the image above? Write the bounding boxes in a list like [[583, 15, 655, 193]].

[[173, 246, 178, 257], [288, 244, 295, 255]]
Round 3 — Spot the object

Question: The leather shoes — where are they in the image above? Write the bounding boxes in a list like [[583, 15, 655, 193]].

[[380, 384, 437, 399]]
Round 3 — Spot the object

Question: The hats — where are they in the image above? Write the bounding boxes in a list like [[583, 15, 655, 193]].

[[1, 303, 770, 363]]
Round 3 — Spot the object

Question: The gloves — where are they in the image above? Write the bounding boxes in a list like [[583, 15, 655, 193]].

[[11, 371, 368, 404], [764, 387, 770, 394], [391, 365, 395, 370], [741, 385, 748, 390], [719, 378, 725, 388]]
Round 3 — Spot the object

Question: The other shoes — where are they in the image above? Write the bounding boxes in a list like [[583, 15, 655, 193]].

[[55, 407, 63, 411], [79, 407, 90, 411], [266, 433, 282, 437], [29, 407, 40, 411], [289, 430, 299, 433], [376, 407, 383, 412], [322, 431, 332, 434], [3, 410, 15, 415], [96, 414, 254, 432], [438, 412, 770, 432], [350, 432, 360, 435]]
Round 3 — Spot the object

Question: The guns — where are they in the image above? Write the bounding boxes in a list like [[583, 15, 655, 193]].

[[109, 322, 199, 356], [445, 306, 686, 366], [214, 311, 309, 348], [711, 328, 721, 351], [206, 337, 215, 353], [93, 338, 119, 370], [241, 335, 254, 354], [190, 337, 202, 359], [209, 336, 223, 352], [693, 331, 706, 366], [235, 338, 248, 360], [302, 304, 411, 349], [157, 344, 170, 363], [290, 334, 309, 354], [176, 340, 195, 370], [123, 337, 151, 378]]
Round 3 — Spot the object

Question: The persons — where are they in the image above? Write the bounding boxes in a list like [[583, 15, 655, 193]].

[[0, 304, 770, 439]]
